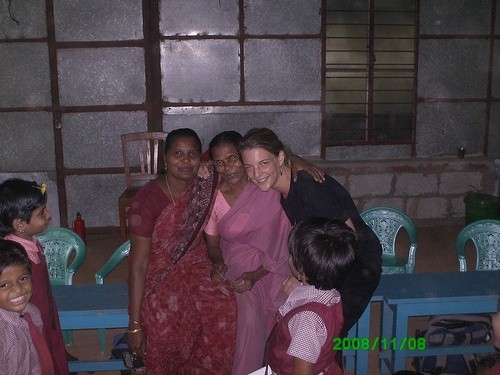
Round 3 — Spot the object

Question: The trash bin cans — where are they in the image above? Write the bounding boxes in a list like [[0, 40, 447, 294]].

[[465, 192, 500, 227]]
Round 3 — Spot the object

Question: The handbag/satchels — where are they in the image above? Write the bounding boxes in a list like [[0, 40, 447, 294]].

[[122, 349, 146, 371], [110, 333, 128, 360]]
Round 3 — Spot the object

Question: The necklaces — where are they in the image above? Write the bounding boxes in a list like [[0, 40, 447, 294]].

[[165, 176, 177, 207]]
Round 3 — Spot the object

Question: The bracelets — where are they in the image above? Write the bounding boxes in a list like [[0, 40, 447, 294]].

[[129, 319, 143, 326], [127, 327, 144, 336]]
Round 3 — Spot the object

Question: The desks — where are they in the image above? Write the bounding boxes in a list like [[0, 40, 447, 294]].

[[377, 269, 500, 375], [45, 278, 382, 375]]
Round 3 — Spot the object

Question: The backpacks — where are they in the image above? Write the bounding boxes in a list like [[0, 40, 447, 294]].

[[414, 314, 495, 374]]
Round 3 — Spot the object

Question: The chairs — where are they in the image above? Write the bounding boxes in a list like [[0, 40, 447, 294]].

[[358, 205, 420, 279], [34, 225, 88, 345], [94, 237, 130, 357], [116, 130, 170, 242], [455, 220, 500, 271]]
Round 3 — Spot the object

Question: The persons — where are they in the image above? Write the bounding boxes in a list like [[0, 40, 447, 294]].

[[204, 131, 291, 375], [239, 129, 382, 372], [265, 216, 357, 374], [127, 129, 325, 374], [0, 178, 70, 375], [0, 240, 55, 375]]
